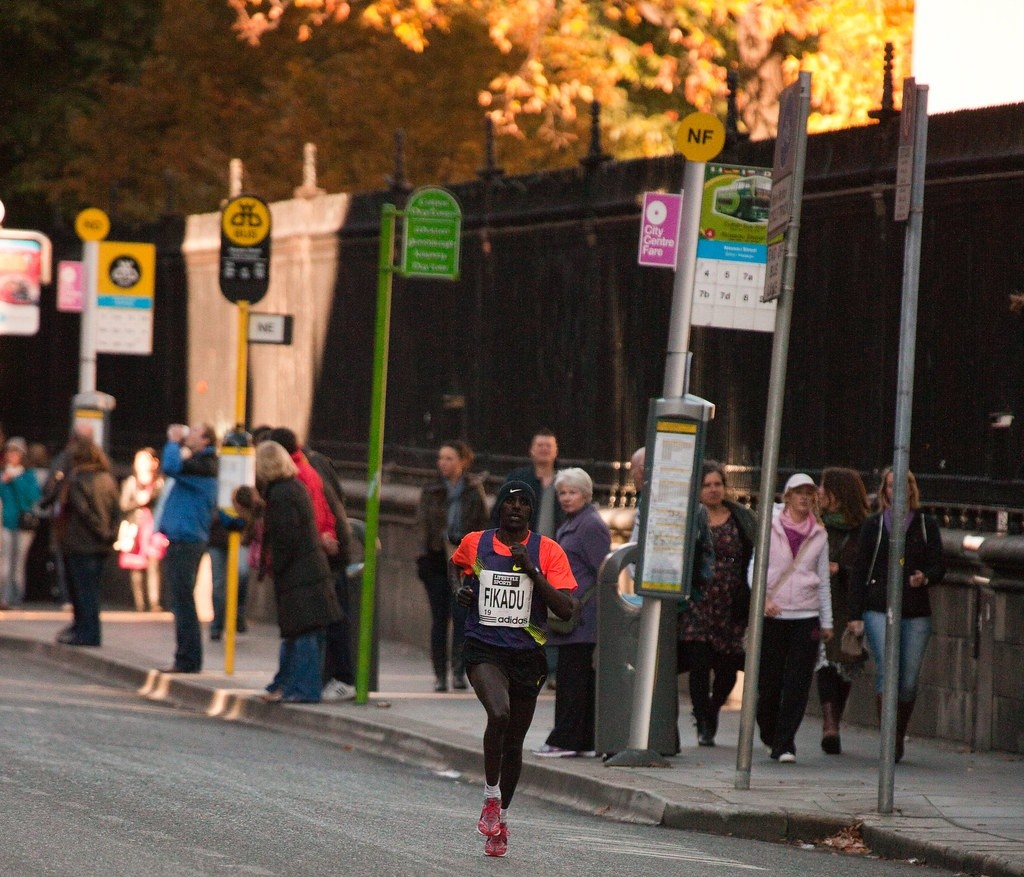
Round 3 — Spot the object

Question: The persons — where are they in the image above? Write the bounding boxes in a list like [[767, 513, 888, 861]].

[[536, 467, 610, 757], [627, 447, 714, 617], [0, 420, 379, 704], [446, 480, 579, 857], [846, 465, 946, 767], [812, 466, 867, 753], [746, 473, 833, 763], [412, 439, 490, 693], [679, 464, 760, 747], [504, 430, 570, 686]]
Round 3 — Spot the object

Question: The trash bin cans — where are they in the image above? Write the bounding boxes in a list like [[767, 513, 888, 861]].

[[347, 520, 379, 693], [595, 542, 678, 756]]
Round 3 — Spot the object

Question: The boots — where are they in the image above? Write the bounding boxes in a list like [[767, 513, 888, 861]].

[[821, 701, 842, 754], [713, 672, 736, 735], [894, 698, 916, 762], [691, 695, 712, 745]]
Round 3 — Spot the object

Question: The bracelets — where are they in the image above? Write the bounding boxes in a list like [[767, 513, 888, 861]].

[[528, 567, 540, 577]]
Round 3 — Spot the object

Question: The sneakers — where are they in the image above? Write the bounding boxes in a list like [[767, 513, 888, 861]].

[[477, 797, 502, 837], [320, 678, 356, 700], [484, 822, 511, 857]]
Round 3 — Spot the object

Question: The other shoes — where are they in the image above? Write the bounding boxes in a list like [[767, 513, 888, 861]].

[[780, 752, 797, 763], [56, 627, 98, 646], [531, 742, 596, 757], [453, 675, 467, 688], [435, 678, 446, 691]]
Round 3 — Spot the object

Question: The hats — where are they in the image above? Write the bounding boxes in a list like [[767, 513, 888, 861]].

[[497, 480, 535, 521], [785, 473, 817, 493]]
